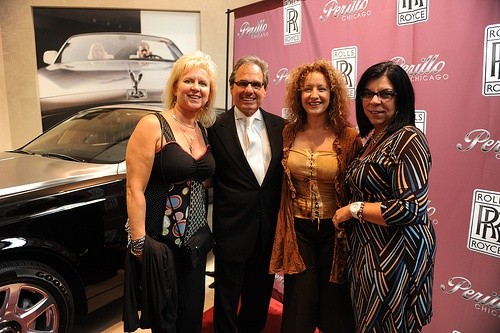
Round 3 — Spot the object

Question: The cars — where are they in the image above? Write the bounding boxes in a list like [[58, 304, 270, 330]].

[[35, 31, 188, 119], [0, 101, 230, 333]]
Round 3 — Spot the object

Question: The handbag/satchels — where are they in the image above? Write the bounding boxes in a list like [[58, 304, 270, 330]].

[[185, 223, 216, 269]]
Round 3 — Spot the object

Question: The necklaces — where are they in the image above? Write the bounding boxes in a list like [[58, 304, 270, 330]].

[[172, 110, 198, 154], [371, 125, 387, 147]]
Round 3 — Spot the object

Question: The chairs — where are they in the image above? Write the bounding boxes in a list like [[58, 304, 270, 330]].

[[115, 44, 139, 59]]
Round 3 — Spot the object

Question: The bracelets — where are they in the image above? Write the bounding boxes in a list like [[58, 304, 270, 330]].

[[131, 237, 145, 256], [359, 202, 365, 223]]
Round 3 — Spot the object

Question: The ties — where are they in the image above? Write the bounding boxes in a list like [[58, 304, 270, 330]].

[[243, 116, 264, 186]]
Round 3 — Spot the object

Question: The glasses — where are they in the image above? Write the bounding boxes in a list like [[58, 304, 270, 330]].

[[359, 89, 397, 103], [233, 79, 264, 90]]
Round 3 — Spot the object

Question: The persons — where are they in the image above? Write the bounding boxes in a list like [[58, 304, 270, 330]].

[[203, 56, 288, 333], [124, 48, 218, 333], [136, 42, 150, 59], [88, 43, 114, 60], [331, 61, 436, 333], [270, 62, 362, 333]]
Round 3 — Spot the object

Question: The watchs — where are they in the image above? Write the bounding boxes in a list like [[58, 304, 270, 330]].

[[349, 201, 363, 219]]
